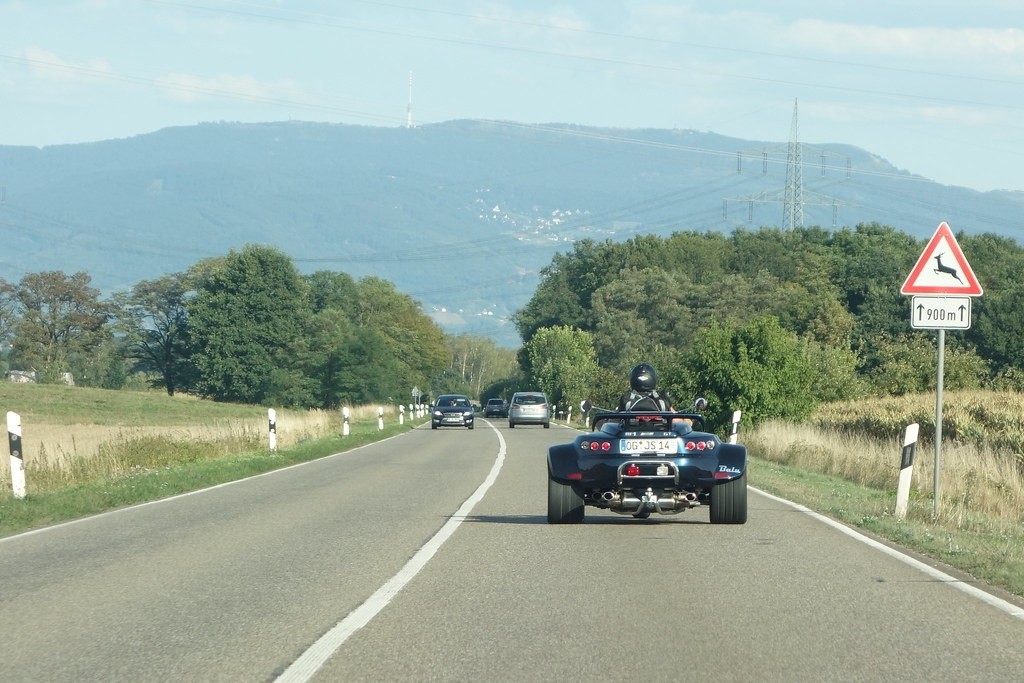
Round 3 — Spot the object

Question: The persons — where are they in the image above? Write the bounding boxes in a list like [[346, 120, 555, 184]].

[[614, 364, 691, 426]]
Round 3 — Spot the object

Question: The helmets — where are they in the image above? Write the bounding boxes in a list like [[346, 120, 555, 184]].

[[630, 365, 656, 392]]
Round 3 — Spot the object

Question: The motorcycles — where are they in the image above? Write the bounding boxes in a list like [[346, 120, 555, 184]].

[[547, 398, 748, 526]]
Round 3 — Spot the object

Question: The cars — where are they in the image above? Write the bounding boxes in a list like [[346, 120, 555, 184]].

[[508, 392, 551, 428], [485, 399, 508, 418], [429, 395, 477, 430]]
